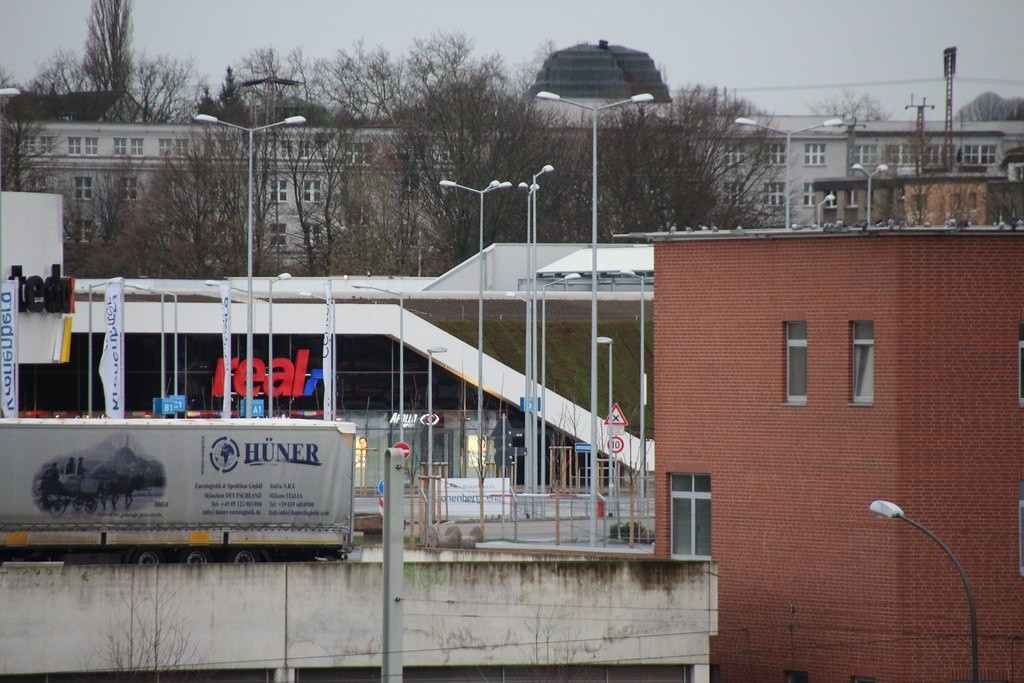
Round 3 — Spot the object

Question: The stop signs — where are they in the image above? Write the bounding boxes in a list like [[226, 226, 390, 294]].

[[393, 442, 410, 460]]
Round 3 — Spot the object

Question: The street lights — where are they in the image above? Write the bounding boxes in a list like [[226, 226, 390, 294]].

[[440, 180, 513, 487], [869, 500, 979, 683], [197, 113, 306, 418], [536, 90, 654, 545], [852, 162, 890, 225], [125, 282, 166, 417], [516, 182, 541, 515], [539, 272, 581, 516], [427, 347, 444, 544], [88, 276, 123, 418], [817, 195, 836, 230], [734, 117, 844, 229], [204, 279, 274, 417], [301, 291, 335, 421], [595, 334, 612, 545], [351, 281, 404, 446], [268, 273, 291, 421], [533, 164, 554, 517]]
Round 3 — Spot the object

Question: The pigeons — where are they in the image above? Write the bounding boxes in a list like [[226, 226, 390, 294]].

[[666, 215, 1024, 234]]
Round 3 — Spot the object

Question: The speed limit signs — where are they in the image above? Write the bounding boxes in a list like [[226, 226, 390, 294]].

[[606, 437, 624, 454]]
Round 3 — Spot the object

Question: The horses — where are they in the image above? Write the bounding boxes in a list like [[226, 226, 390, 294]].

[[98, 469, 142, 512]]
